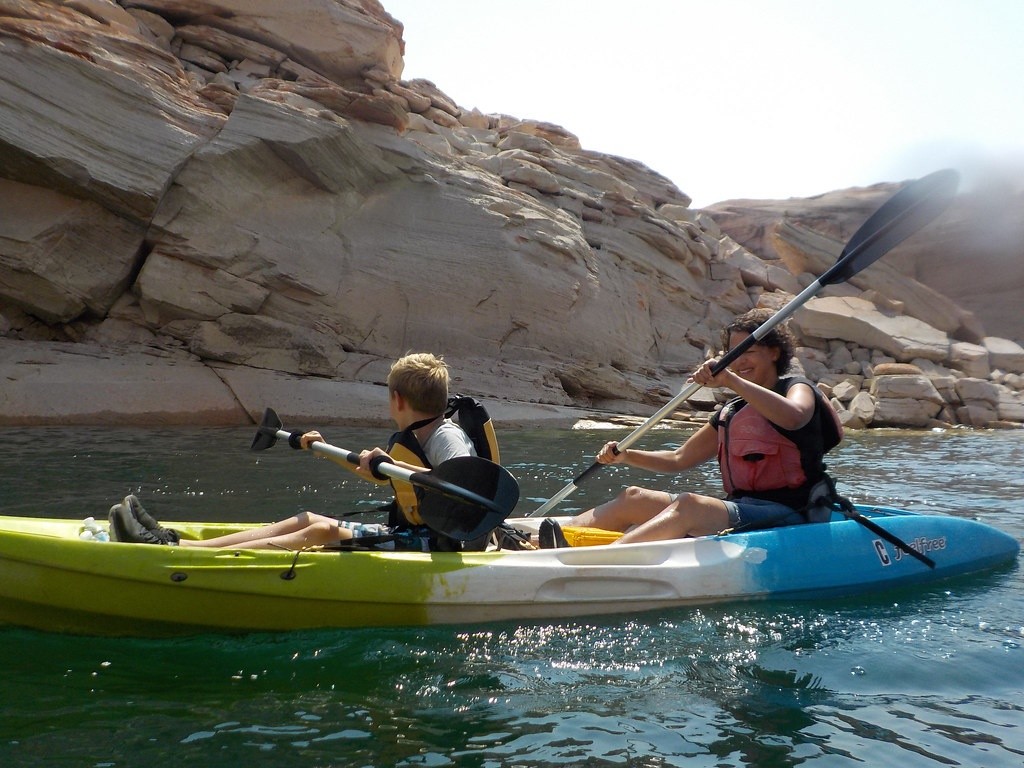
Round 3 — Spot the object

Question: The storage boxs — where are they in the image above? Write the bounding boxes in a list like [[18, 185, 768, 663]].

[[559, 523, 625, 548]]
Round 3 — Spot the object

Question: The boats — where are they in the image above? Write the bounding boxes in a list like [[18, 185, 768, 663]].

[[0, 505, 1019, 630]]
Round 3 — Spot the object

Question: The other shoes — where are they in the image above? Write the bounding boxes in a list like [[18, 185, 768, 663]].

[[537, 517, 572, 550]]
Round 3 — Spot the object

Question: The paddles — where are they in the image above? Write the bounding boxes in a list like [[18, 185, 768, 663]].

[[250, 406, 520, 543], [525, 169, 962, 519]]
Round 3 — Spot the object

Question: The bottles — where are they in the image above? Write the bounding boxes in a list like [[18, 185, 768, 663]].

[[79, 531, 101, 541], [83, 517, 106, 535]]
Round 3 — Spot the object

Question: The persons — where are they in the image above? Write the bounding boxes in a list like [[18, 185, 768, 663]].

[[108, 353, 500, 552], [489, 309, 834, 551]]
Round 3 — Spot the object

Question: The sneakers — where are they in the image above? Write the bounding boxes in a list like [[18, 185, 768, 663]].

[[108, 503, 169, 546], [123, 494, 180, 546]]
